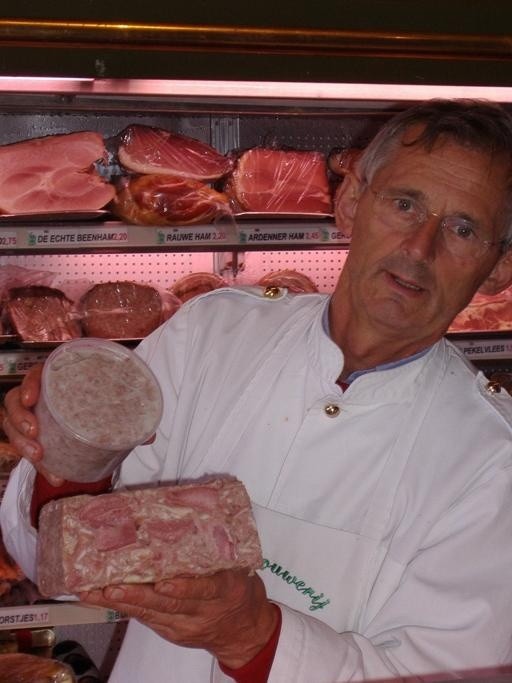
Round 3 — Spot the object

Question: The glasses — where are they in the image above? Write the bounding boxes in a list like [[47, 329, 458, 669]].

[[365, 174, 499, 260]]
